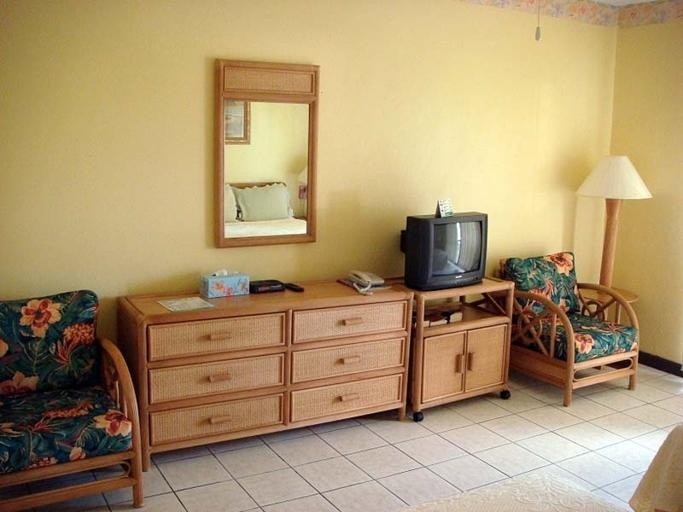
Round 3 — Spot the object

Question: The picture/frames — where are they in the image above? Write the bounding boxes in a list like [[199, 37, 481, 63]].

[[223, 99, 252, 146]]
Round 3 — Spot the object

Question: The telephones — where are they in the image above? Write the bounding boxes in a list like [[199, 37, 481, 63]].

[[348, 270, 384, 286]]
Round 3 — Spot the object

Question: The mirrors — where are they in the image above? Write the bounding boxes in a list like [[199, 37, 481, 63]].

[[213, 58, 319, 249]]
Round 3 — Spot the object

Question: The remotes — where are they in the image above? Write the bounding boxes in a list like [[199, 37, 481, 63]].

[[284, 283, 304, 292]]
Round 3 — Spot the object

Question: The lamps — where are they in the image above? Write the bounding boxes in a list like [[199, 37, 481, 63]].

[[576, 154, 654, 319]]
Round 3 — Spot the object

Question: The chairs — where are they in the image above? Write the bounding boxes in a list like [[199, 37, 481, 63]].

[[491, 249, 638, 410], [0, 288, 145, 511]]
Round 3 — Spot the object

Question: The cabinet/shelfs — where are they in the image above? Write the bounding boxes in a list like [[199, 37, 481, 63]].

[[117, 278, 413, 471], [393, 276, 514, 422]]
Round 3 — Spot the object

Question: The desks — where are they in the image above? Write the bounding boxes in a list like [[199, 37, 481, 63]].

[[580, 285, 638, 322]]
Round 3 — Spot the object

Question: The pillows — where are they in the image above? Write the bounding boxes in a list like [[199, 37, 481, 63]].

[[224, 183, 236, 223], [236, 184, 293, 222]]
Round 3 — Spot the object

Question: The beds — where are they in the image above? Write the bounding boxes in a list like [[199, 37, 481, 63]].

[[225, 180, 305, 236]]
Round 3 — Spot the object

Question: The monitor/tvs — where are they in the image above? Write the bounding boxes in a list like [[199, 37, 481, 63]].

[[400, 212, 488, 291]]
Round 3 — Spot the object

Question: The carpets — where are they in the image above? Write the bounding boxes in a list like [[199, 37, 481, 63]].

[[395, 473, 628, 510]]
